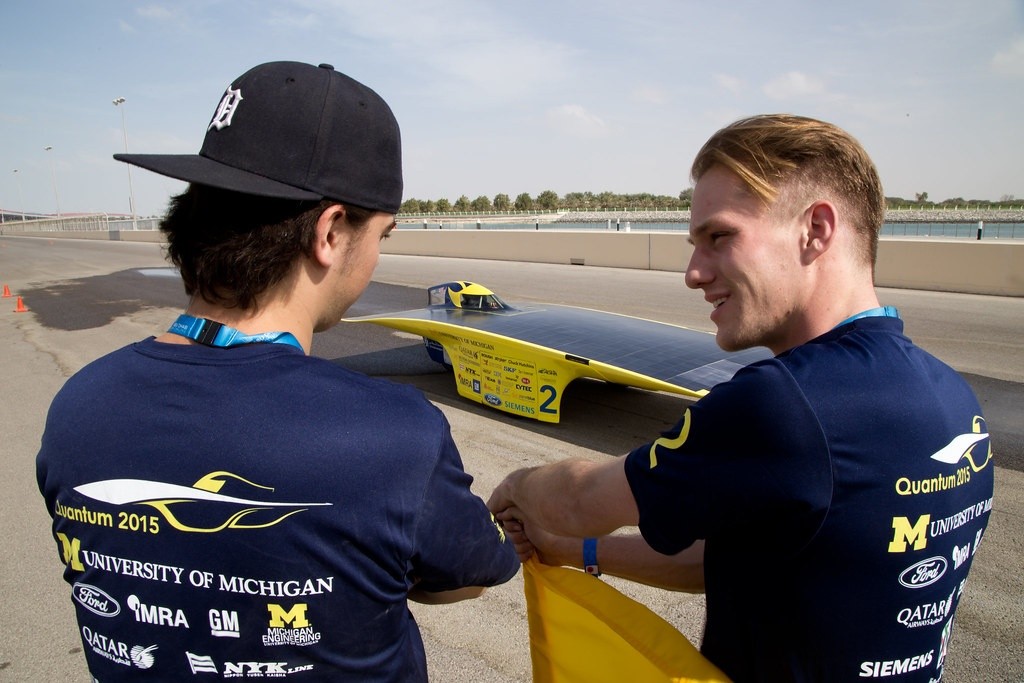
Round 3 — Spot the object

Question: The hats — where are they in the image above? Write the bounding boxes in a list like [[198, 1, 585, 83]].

[[113, 61, 403, 213]]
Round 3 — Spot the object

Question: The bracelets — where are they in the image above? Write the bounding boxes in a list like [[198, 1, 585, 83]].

[[582, 537, 601, 578]]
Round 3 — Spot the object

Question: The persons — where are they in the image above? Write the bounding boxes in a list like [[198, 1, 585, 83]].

[[36, 60, 521, 683], [487, 114, 994, 683]]
